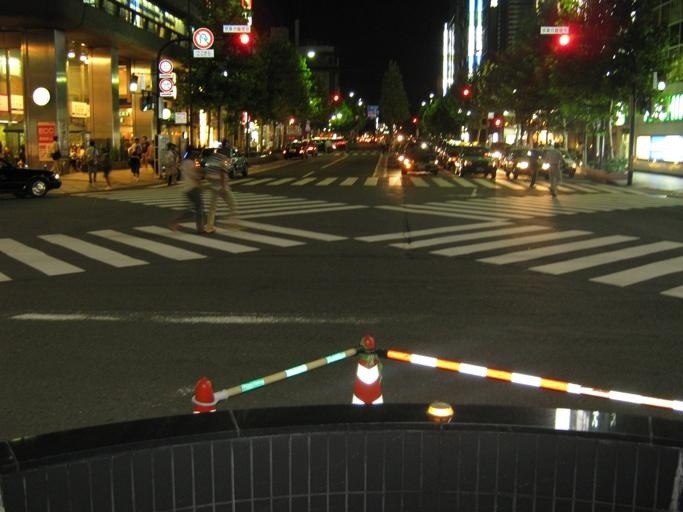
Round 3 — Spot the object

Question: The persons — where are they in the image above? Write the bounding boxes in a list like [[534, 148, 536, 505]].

[[0, 136, 179, 192], [167, 147, 207, 237], [577, 141, 583, 159], [528, 142, 539, 189], [546, 142, 566, 198], [204, 148, 240, 235]]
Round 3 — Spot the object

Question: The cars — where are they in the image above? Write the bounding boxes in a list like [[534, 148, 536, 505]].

[[0, 159, 63, 197], [282, 135, 345, 159], [195, 146, 249, 178], [389, 137, 497, 179], [504, 147, 576, 179]]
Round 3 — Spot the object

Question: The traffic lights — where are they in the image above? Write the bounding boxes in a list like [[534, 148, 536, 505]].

[[559, 35, 571, 46], [236, 34, 251, 46]]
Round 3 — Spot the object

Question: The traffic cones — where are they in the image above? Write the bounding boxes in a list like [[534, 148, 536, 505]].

[[350, 338, 384, 405]]
